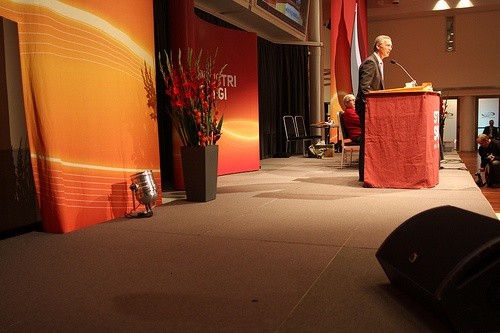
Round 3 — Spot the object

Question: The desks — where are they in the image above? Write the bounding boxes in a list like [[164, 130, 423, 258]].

[[309, 123, 340, 145]]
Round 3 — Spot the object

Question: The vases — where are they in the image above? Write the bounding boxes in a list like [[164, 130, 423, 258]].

[[180, 144, 219, 203]]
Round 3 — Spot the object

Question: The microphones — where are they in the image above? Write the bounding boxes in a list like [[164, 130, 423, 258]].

[[391, 60, 418, 86]]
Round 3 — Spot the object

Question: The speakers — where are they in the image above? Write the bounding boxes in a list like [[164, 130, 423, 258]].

[[375, 204, 500, 333]]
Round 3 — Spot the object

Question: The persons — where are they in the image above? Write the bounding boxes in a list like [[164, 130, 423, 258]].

[[354, 34, 392, 182], [476, 134, 500, 188], [481, 119, 497, 151], [341, 94, 365, 144]]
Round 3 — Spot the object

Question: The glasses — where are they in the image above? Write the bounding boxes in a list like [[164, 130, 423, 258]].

[[347, 99, 355, 103]]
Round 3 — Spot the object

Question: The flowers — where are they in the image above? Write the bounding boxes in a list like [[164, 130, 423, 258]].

[[157, 46, 228, 147]]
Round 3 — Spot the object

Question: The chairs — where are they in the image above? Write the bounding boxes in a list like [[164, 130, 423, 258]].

[[337, 111, 360, 169], [294, 115, 322, 154], [283, 115, 315, 158]]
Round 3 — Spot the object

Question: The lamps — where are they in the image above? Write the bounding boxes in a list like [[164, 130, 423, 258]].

[[124, 169, 159, 219]]
